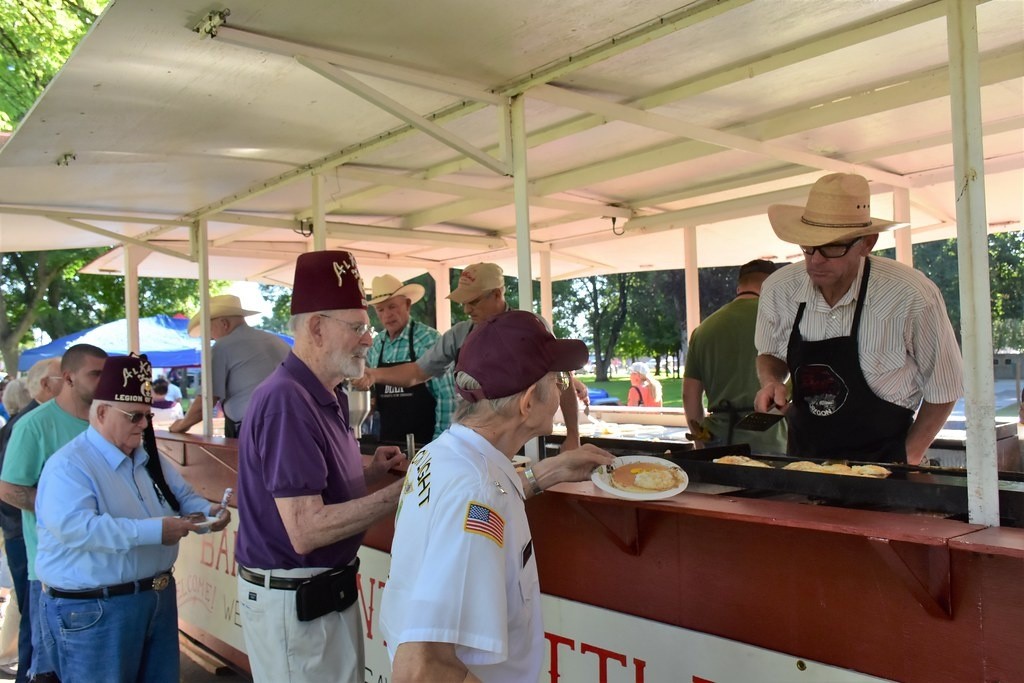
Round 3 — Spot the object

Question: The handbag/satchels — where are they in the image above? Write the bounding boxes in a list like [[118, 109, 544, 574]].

[[638, 400, 644, 407]]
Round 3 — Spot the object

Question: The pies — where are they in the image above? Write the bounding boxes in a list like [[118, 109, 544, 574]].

[[610, 461, 682, 493]]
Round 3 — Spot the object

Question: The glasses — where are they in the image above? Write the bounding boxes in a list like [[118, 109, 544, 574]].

[[106, 404, 154, 424], [799, 235, 863, 258], [550, 371, 570, 392], [461, 290, 493, 306], [317, 314, 376, 337]]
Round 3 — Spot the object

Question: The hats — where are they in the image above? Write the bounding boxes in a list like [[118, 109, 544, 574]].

[[767, 173, 911, 246], [291, 250, 368, 315], [444, 262, 505, 303], [739, 259, 777, 278], [629, 362, 648, 374], [93, 356, 154, 405], [453, 310, 589, 403], [188, 295, 261, 338], [367, 274, 425, 308]]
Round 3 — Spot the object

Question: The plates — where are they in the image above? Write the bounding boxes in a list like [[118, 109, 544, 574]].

[[511, 455, 531, 468], [173, 515, 219, 527], [591, 455, 688, 500]]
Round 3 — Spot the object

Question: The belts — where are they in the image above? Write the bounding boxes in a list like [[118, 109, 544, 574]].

[[238, 557, 360, 590], [42, 568, 173, 599]]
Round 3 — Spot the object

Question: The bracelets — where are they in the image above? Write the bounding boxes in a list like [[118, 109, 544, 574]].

[[524, 466, 543, 496]]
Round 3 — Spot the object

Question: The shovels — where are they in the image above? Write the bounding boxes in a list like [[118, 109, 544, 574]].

[[734, 402, 786, 432]]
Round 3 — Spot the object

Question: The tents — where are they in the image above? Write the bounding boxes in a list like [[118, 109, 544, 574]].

[[18, 315, 295, 398]]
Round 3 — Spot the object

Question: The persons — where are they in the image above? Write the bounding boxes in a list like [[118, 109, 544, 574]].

[[0, 343, 233, 683], [168, 295, 291, 438], [682, 259, 789, 455], [233, 249, 407, 683], [349, 262, 592, 468], [627, 362, 663, 408], [753, 172, 964, 474], [378, 310, 590, 683]]
